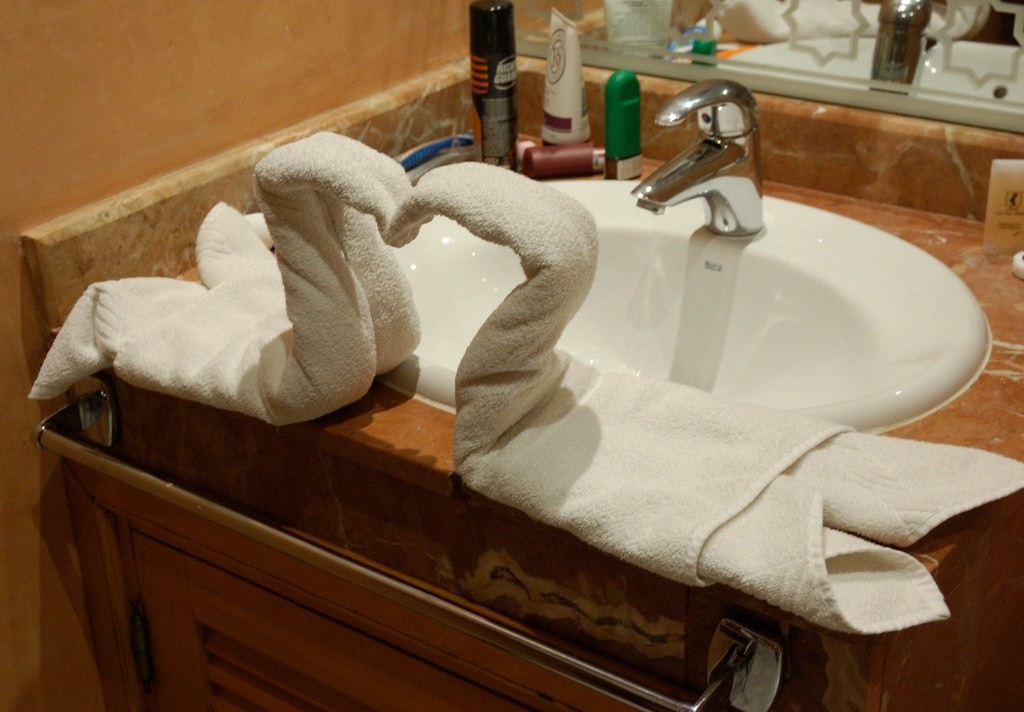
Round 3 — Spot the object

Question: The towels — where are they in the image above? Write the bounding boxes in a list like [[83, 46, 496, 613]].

[[713, 0, 1024, 44], [388, 163, 1021, 634], [26, 129, 411, 422]]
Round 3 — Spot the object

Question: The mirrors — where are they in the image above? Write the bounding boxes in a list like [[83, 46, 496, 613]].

[[513, 0, 1024, 134]]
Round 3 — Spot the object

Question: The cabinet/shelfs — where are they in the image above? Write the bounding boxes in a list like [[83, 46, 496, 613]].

[[32, 360, 1024, 712]]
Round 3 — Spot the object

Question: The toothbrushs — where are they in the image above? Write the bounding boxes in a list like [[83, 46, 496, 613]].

[[383, 133, 474, 170]]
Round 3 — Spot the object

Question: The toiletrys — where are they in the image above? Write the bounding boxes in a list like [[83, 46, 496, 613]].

[[521, 144, 605, 181], [539, 7, 593, 144], [606, 0, 669, 61], [605, 67, 643, 179], [670, 16, 720, 54], [983, 155, 1024, 256], [470, 2, 518, 170], [688, 36, 716, 68]]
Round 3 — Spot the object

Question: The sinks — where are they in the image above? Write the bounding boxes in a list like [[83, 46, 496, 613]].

[[388, 178, 991, 423], [722, 36, 1023, 130]]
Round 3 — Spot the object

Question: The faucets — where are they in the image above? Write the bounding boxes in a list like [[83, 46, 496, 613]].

[[629, 80, 769, 238], [870, 2, 933, 98]]
